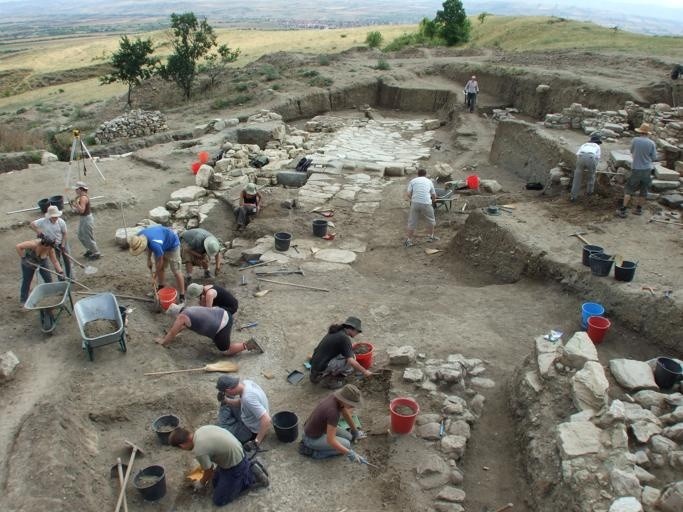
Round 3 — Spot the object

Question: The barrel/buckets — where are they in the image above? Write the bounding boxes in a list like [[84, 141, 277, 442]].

[[313, 220, 328, 237], [586, 316, 611, 344], [271, 411, 300, 442], [588, 253, 615, 277], [582, 302, 605, 329], [274, 232, 293, 251], [615, 260, 638, 281], [467, 176, 478, 189], [152, 415, 180, 446], [133, 466, 166, 500], [582, 245, 604, 267], [158, 287, 177, 310], [389, 397, 419, 433], [352, 341, 375, 369], [193, 151, 208, 175], [654, 358, 683, 390]]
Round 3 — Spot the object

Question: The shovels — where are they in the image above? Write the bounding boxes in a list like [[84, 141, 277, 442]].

[[55, 244, 99, 276], [114, 439, 144, 512], [642, 285, 657, 295], [662, 286, 673, 297], [151, 271, 161, 316]]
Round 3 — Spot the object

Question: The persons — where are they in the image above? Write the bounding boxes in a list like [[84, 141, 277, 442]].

[[185, 280, 239, 315], [294, 382, 365, 466], [169, 425, 271, 504], [401, 169, 440, 248], [232, 182, 262, 233], [130, 223, 186, 303], [16, 235, 68, 304], [159, 303, 267, 357], [212, 374, 273, 450], [178, 227, 222, 283], [462, 75, 479, 113], [67, 182, 103, 260], [615, 122, 659, 219], [567, 131, 604, 202], [305, 315, 373, 387], [29, 205, 75, 283]]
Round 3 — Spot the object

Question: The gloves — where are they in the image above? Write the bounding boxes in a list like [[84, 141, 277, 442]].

[[217, 392, 225, 402], [58, 271, 66, 281], [243, 439, 259, 452], [59, 246, 69, 258], [347, 449, 368, 465], [215, 269, 223, 276], [351, 430, 368, 444]]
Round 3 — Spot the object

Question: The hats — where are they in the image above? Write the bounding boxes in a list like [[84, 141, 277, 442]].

[[165, 303, 185, 317], [129, 235, 148, 256], [343, 315, 363, 334], [203, 236, 220, 257], [45, 205, 63, 218], [333, 384, 363, 408], [72, 182, 88, 191], [184, 283, 204, 299], [216, 376, 240, 390], [42, 236, 56, 245]]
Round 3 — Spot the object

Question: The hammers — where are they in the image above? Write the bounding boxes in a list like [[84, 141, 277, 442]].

[[239, 274, 248, 287], [256, 266, 306, 277], [569, 231, 590, 245], [438, 415, 447, 436], [290, 245, 300, 254]]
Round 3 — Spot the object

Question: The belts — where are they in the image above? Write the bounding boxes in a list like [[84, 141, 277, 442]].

[[219, 453, 246, 471]]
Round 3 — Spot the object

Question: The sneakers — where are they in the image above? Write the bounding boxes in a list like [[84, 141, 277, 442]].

[[146, 291, 155, 298], [250, 463, 270, 488], [252, 457, 268, 476], [245, 336, 265, 353], [426, 237, 433, 243], [180, 298, 187, 306], [184, 276, 193, 284], [204, 271, 215, 279], [405, 240, 414, 247]]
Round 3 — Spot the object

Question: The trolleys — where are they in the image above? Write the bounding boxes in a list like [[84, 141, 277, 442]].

[[432, 188, 457, 213], [23, 281, 72, 338], [582, 170, 623, 198], [74, 292, 129, 362]]
